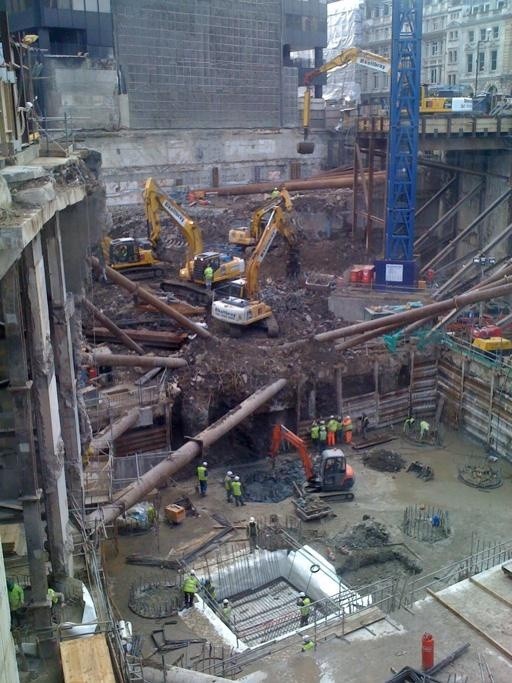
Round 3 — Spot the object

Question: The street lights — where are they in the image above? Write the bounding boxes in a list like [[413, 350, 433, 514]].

[[475, 24, 499, 102], [474, 257, 497, 329]]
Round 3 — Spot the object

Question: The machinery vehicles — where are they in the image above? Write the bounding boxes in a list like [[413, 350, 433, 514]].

[[142, 174, 253, 309], [265, 420, 356, 502], [228, 187, 303, 258], [298, 49, 475, 154], [98, 231, 168, 286], [208, 204, 304, 339], [452, 298, 511, 357]]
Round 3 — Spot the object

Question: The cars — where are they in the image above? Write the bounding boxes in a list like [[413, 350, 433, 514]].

[[434, 82, 472, 97]]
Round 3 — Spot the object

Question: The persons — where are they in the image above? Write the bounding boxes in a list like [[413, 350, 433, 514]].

[[7, 576, 66, 626], [271, 187, 280, 198], [223, 471, 245, 507], [311, 413, 369, 448], [197, 461, 208, 495], [180, 570, 315, 652], [204, 263, 213, 291], [400, 413, 430, 441]]
